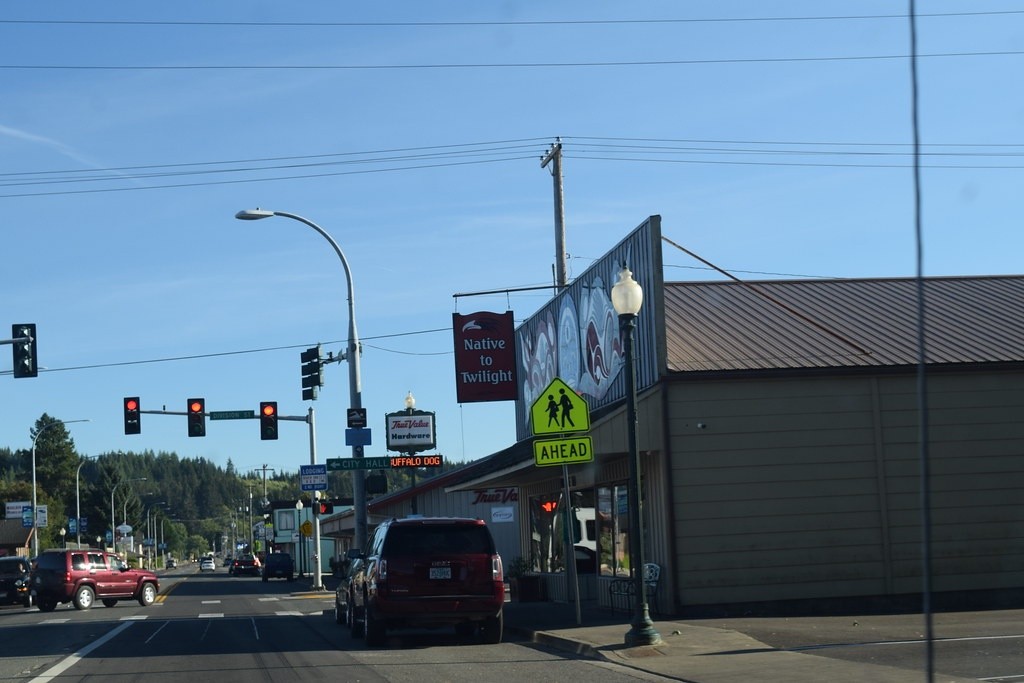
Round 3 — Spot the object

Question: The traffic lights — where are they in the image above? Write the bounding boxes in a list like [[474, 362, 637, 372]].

[[124, 397, 141, 435], [301, 343, 323, 400], [187, 398, 206, 437], [12, 324, 38, 378], [260, 402, 278, 440], [319, 499, 333, 515]]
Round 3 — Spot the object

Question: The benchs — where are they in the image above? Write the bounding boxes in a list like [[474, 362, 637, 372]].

[[609, 563, 661, 614]]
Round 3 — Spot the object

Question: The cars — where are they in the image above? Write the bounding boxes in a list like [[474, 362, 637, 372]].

[[198, 557, 215, 572], [223, 553, 295, 582], [166, 558, 177, 570]]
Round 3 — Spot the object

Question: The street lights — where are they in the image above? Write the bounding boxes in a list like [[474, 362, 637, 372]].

[[154, 508, 171, 570], [296, 498, 305, 579], [124, 493, 153, 538], [161, 514, 176, 568], [236, 207, 368, 557], [148, 502, 166, 570], [404, 391, 417, 517], [76, 453, 123, 549], [112, 478, 147, 553], [611, 260, 661, 645], [33, 419, 90, 561]]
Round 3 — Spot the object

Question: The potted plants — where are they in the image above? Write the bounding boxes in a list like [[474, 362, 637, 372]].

[[505, 556, 531, 601]]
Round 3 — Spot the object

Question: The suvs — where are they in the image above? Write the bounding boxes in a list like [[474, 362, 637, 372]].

[[29, 549, 160, 612], [0, 555, 31, 605], [335, 516, 505, 645]]
